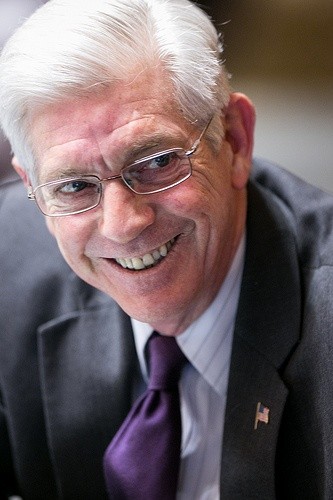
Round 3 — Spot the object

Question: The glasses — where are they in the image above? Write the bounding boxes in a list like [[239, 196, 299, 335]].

[[27, 112, 215, 217]]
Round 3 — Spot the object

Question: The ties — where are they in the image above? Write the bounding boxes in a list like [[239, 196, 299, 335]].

[[102, 336, 186, 500]]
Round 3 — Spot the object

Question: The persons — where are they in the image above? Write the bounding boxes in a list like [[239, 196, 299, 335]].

[[0, 0, 333, 500]]
